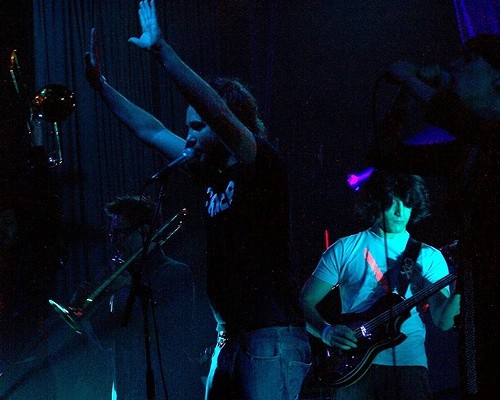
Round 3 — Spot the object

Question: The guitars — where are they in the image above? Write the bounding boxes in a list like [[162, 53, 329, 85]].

[[316, 274, 460, 388]]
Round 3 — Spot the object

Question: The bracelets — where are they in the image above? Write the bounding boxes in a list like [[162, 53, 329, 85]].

[[88, 74, 106, 91], [321, 323, 332, 341]]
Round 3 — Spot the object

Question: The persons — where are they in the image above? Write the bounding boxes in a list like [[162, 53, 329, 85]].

[[70, 196, 222, 400], [299, 166, 464, 400], [390, 33, 500, 400], [82, 0, 314, 400]]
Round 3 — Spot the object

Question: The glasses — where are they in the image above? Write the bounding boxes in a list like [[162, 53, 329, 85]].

[[108, 227, 138, 243]]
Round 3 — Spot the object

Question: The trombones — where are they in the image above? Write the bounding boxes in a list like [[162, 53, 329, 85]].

[[0, 208, 188, 400]]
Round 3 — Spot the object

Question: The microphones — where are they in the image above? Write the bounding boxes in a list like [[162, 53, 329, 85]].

[[386, 61, 457, 84], [146, 147, 196, 184]]
